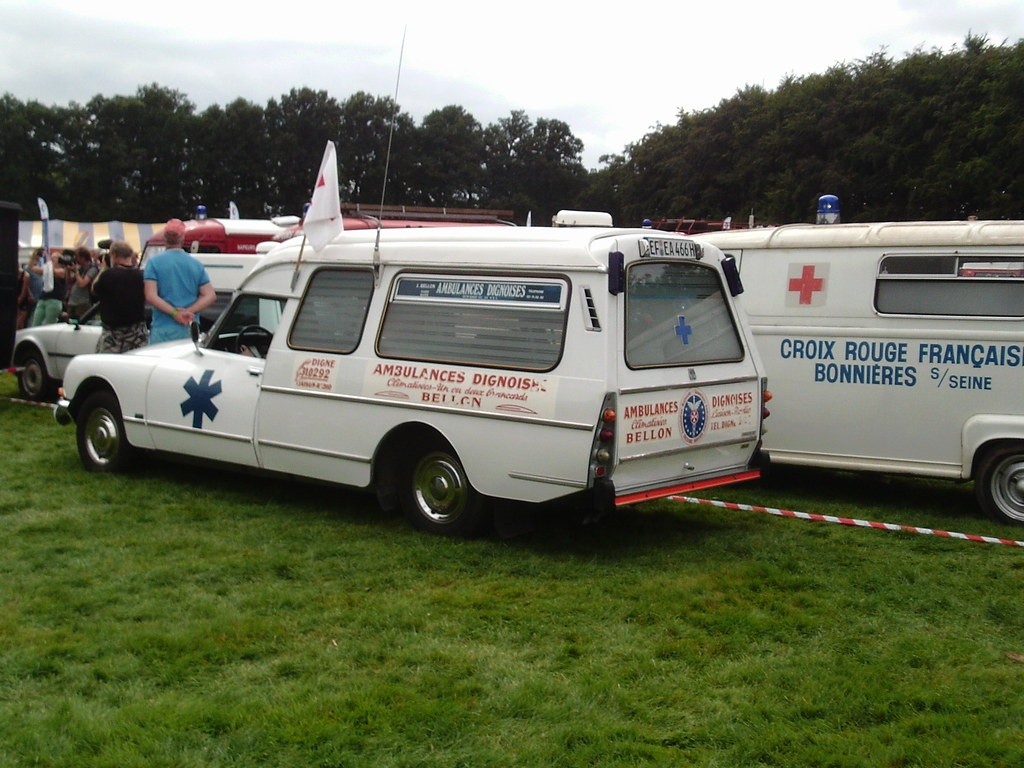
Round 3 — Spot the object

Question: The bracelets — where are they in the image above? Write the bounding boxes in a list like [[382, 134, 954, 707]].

[[169, 309, 177, 318]]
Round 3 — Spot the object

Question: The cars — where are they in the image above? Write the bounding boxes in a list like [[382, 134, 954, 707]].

[[10, 253, 266, 401]]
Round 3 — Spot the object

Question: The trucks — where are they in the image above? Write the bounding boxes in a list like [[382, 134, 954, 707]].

[[139, 199, 517, 270]]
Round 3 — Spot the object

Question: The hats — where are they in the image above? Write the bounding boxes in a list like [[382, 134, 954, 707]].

[[164, 218, 185, 241]]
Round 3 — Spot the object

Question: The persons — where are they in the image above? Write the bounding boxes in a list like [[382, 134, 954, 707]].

[[16, 247, 101, 330], [91, 240, 148, 354], [240, 345, 256, 357], [143, 219, 217, 346]]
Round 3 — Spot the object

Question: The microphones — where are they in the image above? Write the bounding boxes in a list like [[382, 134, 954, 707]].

[[98, 240, 113, 249]]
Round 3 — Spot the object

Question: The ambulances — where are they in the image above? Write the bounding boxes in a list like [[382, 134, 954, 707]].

[[54, 229, 772, 533], [684, 195, 1024, 527]]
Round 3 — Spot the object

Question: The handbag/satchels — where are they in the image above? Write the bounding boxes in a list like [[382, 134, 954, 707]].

[[42, 261, 54, 292]]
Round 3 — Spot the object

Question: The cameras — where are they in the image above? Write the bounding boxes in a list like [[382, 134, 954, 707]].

[[57, 256, 76, 266]]
